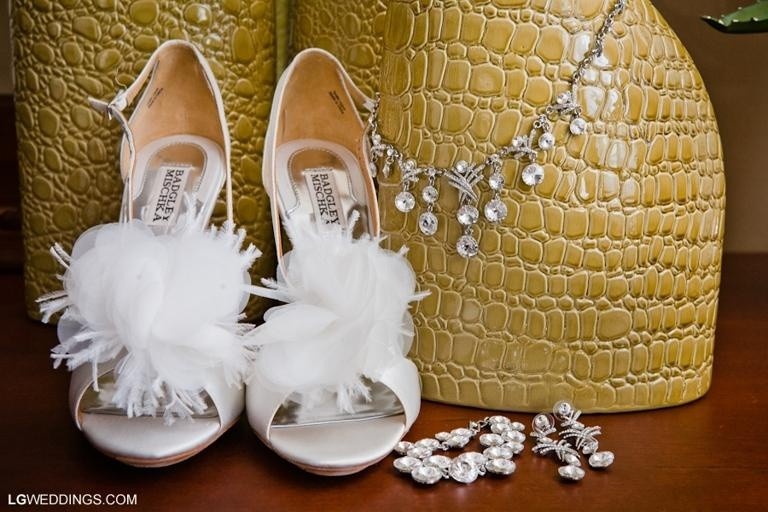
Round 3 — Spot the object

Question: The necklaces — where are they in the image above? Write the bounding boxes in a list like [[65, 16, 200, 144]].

[[365, 0, 632, 261]]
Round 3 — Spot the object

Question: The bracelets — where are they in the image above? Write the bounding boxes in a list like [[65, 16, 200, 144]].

[[391, 414, 526, 486]]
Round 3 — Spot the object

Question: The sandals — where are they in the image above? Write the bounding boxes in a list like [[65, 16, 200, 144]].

[[244, 47, 422, 478], [68, 39, 246, 469]]
